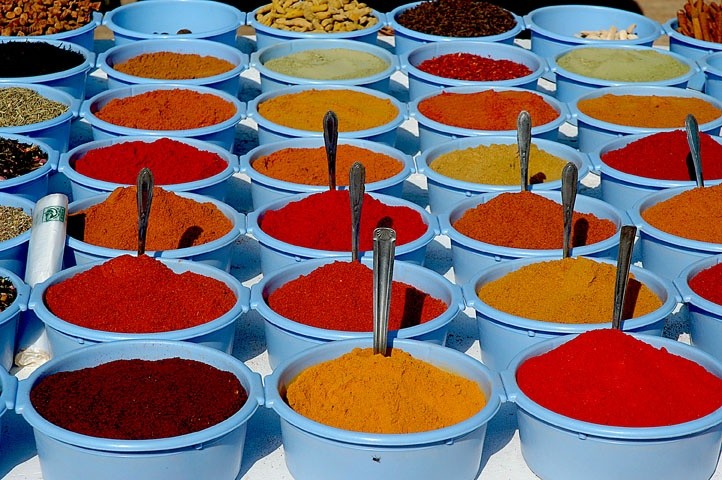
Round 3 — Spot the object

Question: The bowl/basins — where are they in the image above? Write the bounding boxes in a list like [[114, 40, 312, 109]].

[[0, 0, 722, 480]]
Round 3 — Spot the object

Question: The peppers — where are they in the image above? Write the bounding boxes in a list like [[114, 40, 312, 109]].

[[396, 0, 516, 38]]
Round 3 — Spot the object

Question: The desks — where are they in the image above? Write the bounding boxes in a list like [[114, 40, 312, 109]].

[[0, 36, 721, 479]]
